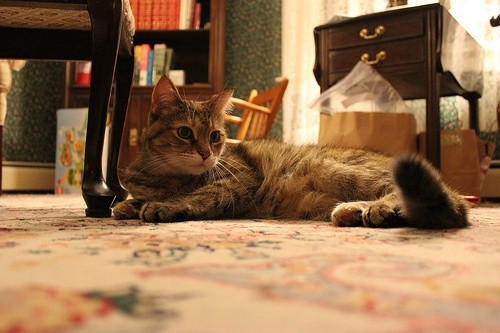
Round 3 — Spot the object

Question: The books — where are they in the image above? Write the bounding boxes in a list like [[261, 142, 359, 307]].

[[133, 42, 174, 87], [128, 0, 202, 31]]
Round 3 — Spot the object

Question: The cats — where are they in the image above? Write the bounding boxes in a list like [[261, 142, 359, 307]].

[[111, 74, 473, 230]]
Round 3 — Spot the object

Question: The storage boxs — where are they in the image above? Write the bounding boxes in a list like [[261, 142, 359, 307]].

[[417, 129, 483, 206], [316, 110, 417, 157]]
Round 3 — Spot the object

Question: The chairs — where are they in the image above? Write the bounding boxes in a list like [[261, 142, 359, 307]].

[[224, 79, 289, 151]]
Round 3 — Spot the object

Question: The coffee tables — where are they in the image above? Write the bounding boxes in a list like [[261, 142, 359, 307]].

[[0, 0, 136, 219]]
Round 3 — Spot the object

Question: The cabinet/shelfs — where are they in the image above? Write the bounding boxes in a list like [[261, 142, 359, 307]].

[[62, 0, 226, 185], [310, 2, 486, 178]]
